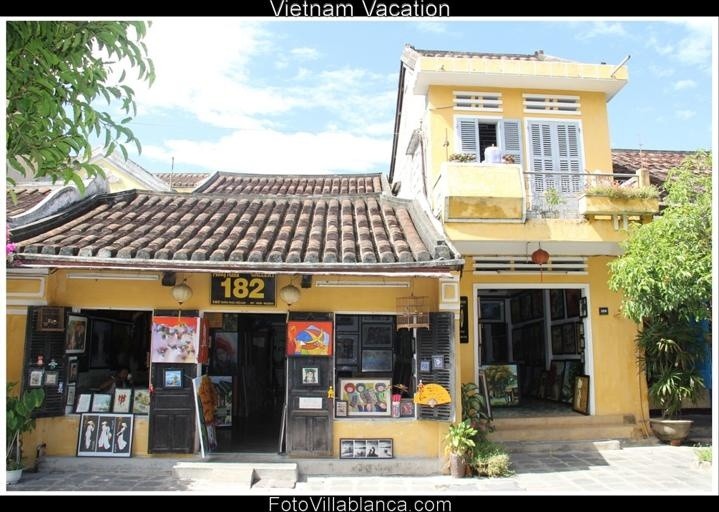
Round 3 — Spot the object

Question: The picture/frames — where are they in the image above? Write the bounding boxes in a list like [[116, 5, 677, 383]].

[[479, 288, 589, 422], [25, 313, 445, 461]]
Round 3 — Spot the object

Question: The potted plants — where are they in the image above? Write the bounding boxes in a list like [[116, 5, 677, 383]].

[[444, 418, 479, 478], [460, 383, 488, 434], [541, 187, 562, 218], [6, 381, 45, 486], [633, 314, 706, 444]]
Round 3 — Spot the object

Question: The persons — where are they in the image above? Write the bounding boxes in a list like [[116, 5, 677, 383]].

[[364, 327, 392, 344], [99, 365, 134, 394], [72, 320, 85, 350], [304, 368, 316, 382], [214, 346, 235, 371]]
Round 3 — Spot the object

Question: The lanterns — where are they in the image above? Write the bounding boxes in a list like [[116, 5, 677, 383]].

[[532, 249, 550, 282]]
